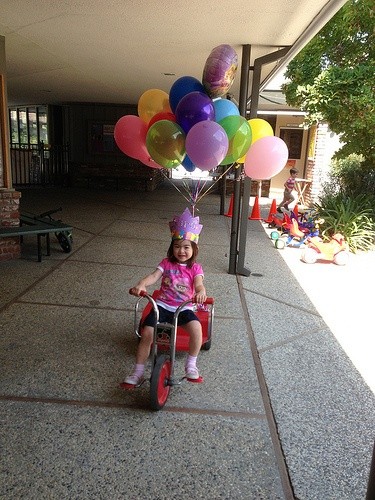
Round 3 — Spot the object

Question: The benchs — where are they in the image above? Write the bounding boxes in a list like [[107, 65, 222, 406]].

[[50, 166, 149, 193], [0, 211, 73, 263]]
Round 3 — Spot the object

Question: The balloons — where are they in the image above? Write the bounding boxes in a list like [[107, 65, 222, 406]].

[[113, 43, 289, 181]]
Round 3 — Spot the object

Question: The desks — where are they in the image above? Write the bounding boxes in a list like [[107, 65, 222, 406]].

[[296, 178, 311, 205]]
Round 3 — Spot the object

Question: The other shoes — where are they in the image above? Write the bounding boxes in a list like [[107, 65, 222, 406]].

[[124, 372, 145, 385], [283, 204, 289, 211], [277, 207, 283, 213], [184, 366, 199, 380]]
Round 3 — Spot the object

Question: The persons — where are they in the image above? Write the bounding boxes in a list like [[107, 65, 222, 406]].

[[277, 167, 299, 213], [123, 208, 207, 385]]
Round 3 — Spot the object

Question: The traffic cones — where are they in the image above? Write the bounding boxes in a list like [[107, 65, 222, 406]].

[[265, 199, 277, 223], [293, 204, 298, 218], [223, 193, 233, 218], [248, 195, 264, 220]]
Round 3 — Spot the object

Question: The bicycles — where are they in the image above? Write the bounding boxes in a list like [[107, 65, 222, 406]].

[[38, 207, 73, 254]]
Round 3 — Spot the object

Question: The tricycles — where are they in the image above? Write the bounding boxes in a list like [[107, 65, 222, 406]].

[[119, 288, 215, 408]]
